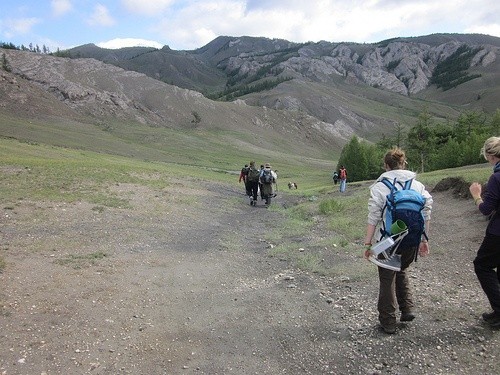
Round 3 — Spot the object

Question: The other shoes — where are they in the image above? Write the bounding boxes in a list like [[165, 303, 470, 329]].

[[400, 313, 415, 321], [381, 325, 396, 333], [489, 317, 500, 326], [482, 311, 498, 323]]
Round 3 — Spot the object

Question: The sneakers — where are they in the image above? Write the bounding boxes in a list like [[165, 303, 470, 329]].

[[368, 253, 402, 272], [379, 236, 395, 260]]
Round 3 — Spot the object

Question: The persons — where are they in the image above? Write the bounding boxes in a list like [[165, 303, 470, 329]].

[[244, 161, 259, 207], [260, 163, 277, 205], [363, 149, 434, 333], [239, 165, 249, 193], [338, 165, 348, 192], [469, 137, 499, 329], [259, 165, 338, 198]]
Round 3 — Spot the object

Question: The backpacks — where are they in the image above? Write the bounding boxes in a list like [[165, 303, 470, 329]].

[[248, 166, 260, 182], [376, 178, 429, 251], [260, 169, 273, 184], [340, 168, 346, 180]]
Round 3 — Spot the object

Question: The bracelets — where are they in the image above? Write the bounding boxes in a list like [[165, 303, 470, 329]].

[[364, 243, 372, 250], [474, 197, 482, 205], [421, 240, 428, 243]]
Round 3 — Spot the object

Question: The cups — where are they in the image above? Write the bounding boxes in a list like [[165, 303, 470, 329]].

[[391, 219, 406, 234]]
